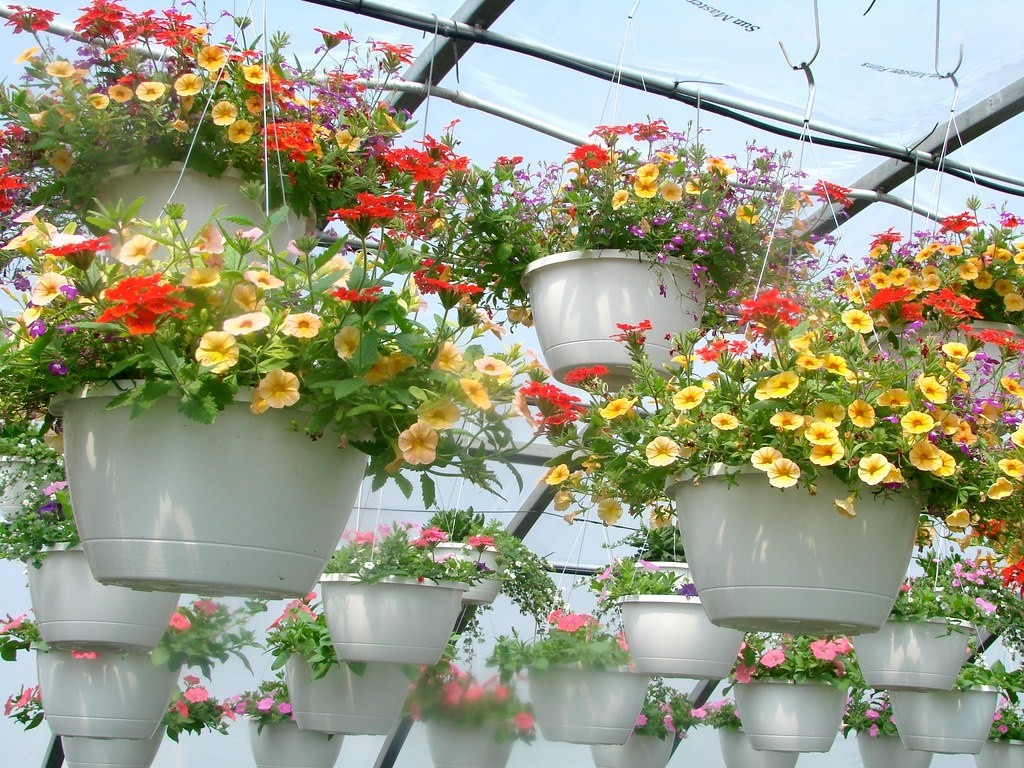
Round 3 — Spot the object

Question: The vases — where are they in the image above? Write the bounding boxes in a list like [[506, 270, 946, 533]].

[[426, 717, 516, 768], [888, 684, 1000, 753], [665, 460, 920, 635], [613, 594, 747, 679], [527, 662, 649, 745], [43, 376, 392, 595], [60, 722, 166, 768], [634, 560, 696, 591], [318, 574, 471, 666], [852, 613, 976, 692], [733, 678, 847, 753], [520, 249, 708, 392], [974, 739, 1023, 768], [32, 642, 186, 742], [83, 153, 315, 272], [25, 542, 180, 655], [719, 726, 799, 767], [428, 541, 507, 604], [284, 651, 410, 734], [857, 729, 933, 768], [590, 731, 676, 767], [866, 322, 1024, 419], [248, 718, 345, 768]]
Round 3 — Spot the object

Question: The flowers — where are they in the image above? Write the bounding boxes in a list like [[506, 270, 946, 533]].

[[0, 0, 1024, 768], [0, 193, 531, 506]]
